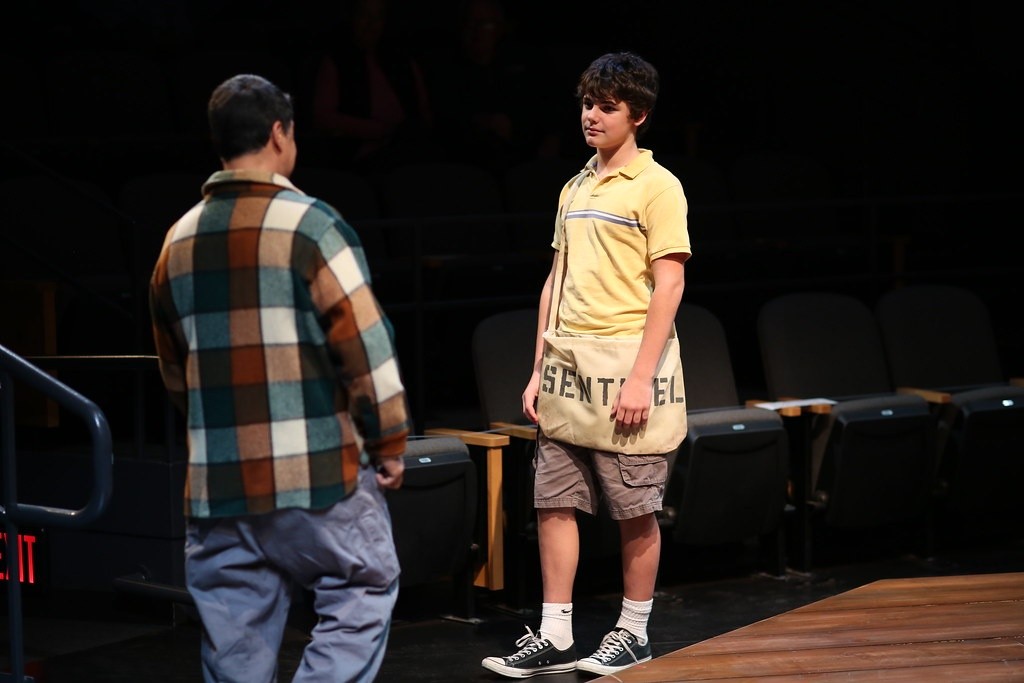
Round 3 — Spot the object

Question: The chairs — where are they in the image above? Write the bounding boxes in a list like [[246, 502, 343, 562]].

[[363, 261, 1023, 640]]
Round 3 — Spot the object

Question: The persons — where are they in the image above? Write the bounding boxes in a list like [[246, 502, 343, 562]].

[[480, 52, 693, 677], [150, 69, 414, 683]]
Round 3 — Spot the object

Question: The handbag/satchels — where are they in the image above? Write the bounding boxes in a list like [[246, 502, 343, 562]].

[[535, 328, 688, 455]]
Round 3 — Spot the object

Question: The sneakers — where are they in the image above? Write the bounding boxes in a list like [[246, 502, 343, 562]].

[[574, 628, 652, 675], [480, 630, 578, 679]]
